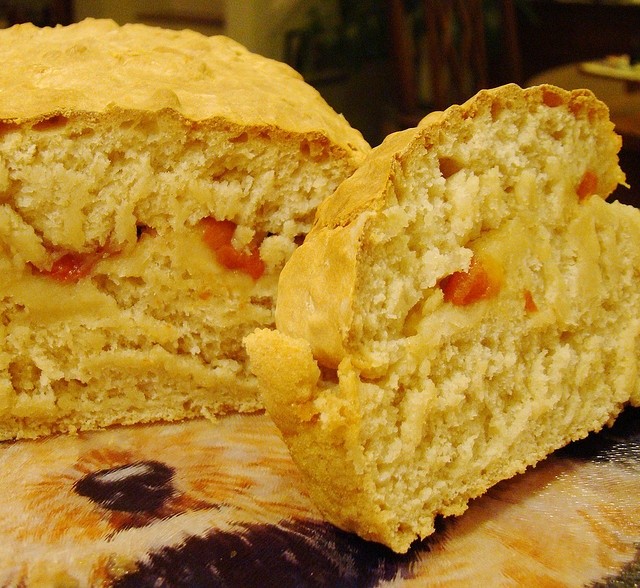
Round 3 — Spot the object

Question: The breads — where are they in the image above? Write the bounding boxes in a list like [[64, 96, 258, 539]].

[[0, 16, 373, 449], [257, 82, 640, 552]]
